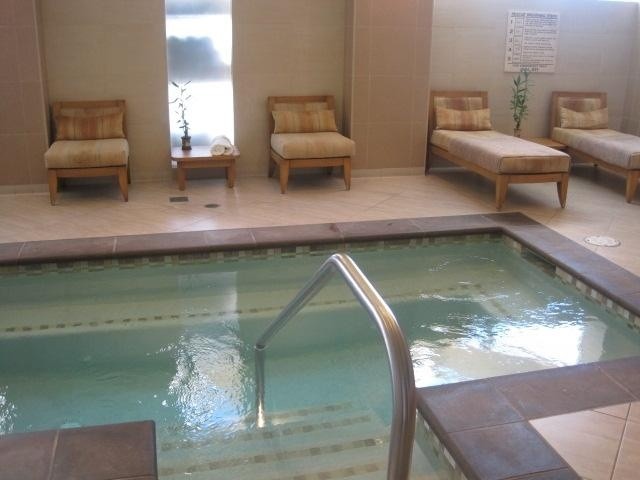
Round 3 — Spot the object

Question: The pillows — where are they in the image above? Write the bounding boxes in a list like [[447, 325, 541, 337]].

[[559, 106, 609, 129], [435, 105, 493, 131]]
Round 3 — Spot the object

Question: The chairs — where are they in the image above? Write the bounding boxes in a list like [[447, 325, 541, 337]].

[[549, 91, 640, 202], [266, 94, 356, 195], [425, 90, 571, 212], [42, 100, 131, 205]]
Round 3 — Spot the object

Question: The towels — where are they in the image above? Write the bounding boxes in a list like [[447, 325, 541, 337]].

[[210, 134, 233, 156]]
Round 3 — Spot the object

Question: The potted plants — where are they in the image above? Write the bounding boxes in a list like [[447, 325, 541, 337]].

[[169, 80, 192, 151], [508, 68, 536, 139]]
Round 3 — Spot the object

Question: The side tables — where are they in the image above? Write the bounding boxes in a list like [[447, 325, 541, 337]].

[[522, 137, 567, 151], [171, 145, 240, 191]]
